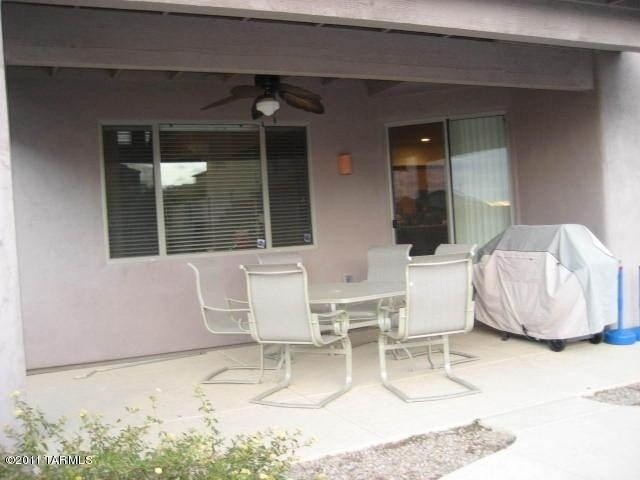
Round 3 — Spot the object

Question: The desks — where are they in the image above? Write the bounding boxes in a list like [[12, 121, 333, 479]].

[[223, 280, 408, 354]]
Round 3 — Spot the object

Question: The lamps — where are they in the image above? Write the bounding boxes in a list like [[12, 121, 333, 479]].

[[256, 98, 281, 116], [335, 152, 353, 177]]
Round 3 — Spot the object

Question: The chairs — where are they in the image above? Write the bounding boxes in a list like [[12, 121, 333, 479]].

[[375, 254, 483, 404], [256, 254, 304, 360], [346, 241, 416, 362], [188, 261, 293, 388], [407, 241, 484, 370], [239, 261, 354, 411]]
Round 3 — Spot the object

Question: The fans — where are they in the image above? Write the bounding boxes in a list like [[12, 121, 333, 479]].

[[200, 73, 323, 121]]
